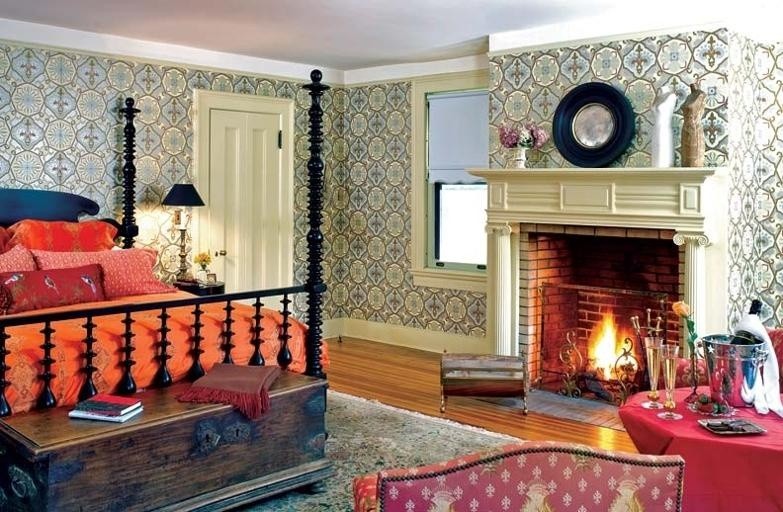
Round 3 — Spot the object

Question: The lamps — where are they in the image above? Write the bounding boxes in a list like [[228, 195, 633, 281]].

[[161, 184, 205, 282]]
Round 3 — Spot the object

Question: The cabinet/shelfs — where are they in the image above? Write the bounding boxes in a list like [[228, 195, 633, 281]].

[[0, 368, 338, 512]]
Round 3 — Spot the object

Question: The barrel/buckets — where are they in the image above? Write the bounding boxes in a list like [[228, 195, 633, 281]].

[[698, 334, 769, 408]]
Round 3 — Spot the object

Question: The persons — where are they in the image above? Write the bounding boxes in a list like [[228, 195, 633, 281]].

[[679, 81, 706, 167], [651, 84, 676, 168]]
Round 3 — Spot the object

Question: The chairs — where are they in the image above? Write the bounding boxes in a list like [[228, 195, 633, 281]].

[[658, 329, 783, 392], [353, 441, 685, 512]]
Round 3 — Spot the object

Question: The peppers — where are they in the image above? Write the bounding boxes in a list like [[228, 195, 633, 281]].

[[699, 393, 729, 413]]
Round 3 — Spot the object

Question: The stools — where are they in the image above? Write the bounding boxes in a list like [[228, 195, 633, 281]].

[[440, 349, 529, 416]]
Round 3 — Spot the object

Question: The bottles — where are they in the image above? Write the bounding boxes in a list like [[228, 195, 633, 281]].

[[729, 300, 763, 345]]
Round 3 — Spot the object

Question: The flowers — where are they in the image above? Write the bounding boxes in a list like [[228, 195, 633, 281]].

[[672, 300, 698, 347], [498, 120, 550, 150], [194, 253, 212, 270]]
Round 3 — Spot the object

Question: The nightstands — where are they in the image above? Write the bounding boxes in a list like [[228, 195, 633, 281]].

[[173, 280, 225, 296]]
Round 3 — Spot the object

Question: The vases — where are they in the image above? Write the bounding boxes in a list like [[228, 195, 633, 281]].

[[685, 341, 699, 403], [196, 264, 210, 284], [505, 147, 525, 169]]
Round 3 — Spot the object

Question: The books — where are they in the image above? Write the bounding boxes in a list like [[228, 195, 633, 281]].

[[67, 405, 144, 423], [74, 391, 143, 414]]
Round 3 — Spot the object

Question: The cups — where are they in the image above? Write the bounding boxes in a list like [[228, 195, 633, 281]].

[[640, 337, 666, 411], [657, 344, 683, 421]]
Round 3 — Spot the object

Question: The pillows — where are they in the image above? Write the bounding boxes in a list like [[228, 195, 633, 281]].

[[0, 243, 38, 272], [5, 219, 118, 253], [29, 248, 179, 299], [0, 263, 106, 316], [0, 227, 9, 254]]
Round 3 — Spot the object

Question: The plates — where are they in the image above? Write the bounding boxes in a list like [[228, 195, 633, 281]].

[[697, 418, 768, 435], [687, 401, 740, 417]]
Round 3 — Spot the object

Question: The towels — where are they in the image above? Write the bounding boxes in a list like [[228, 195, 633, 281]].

[[735, 315, 783, 419]]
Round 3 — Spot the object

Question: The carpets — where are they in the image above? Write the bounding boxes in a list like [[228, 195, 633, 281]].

[[228, 388, 529, 512]]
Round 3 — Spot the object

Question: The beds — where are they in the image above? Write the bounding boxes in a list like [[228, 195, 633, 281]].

[[0, 70, 334, 441]]
